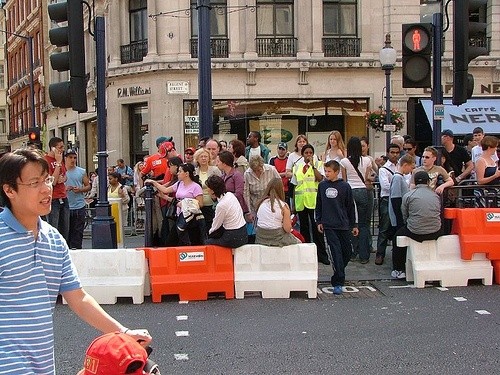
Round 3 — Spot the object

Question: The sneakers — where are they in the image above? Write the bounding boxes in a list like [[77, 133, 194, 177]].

[[333, 286, 342, 294], [391, 269, 406, 279]]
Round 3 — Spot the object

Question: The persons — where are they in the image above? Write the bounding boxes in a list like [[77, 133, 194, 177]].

[[286, 130, 383, 294], [0, 146, 153, 375], [375, 134, 454, 279], [89, 159, 144, 226], [440, 127, 500, 235], [43, 137, 91, 249], [138, 130, 302, 248]]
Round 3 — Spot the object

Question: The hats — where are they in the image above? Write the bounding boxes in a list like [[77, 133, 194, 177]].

[[414, 172, 433, 182], [155, 137, 172, 147], [76, 331, 146, 375], [440, 129, 454, 138], [64, 149, 76, 157], [278, 142, 287, 150], [186, 147, 197, 154]]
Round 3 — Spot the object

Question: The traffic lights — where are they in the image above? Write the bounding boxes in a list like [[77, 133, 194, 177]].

[[452, 0, 488, 107], [28, 127, 40, 142], [47, 0, 89, 114], [402, 22, 434, 89]]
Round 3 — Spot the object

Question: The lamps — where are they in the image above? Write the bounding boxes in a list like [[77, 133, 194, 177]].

[[309, 114, 317, 127]]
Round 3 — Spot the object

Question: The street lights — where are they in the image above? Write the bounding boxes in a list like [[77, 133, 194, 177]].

[[378, 33, 396, 155]]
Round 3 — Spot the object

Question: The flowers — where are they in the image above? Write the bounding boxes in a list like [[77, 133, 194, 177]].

[[364, 108, 405, 131]]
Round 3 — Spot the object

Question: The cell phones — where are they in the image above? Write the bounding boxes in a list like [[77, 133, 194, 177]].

[[51, 146, 56, 152]]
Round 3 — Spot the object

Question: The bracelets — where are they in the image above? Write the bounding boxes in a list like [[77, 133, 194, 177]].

[[56, 163, 62, 166], [121, 327, 129, 333]]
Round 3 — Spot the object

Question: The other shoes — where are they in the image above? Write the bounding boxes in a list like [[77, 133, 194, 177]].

[[360, 257, 368, 263], [351, 250, 360, 260], [375, 255, 383, 265], [322, 258, 331, 265]]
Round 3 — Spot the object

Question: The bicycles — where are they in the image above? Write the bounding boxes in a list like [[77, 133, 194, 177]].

[[83, 198, 97, 229]]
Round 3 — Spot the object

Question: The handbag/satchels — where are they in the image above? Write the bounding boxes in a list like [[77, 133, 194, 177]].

[[166, 180, 180, 219], [288, 183, 294, 197]]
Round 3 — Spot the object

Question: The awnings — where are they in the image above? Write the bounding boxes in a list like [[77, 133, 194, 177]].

[[421, 100, 500, 134]]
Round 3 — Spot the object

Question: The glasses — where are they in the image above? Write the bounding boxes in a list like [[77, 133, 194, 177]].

[[8, 176, 55, 189], [404, 148, 415, 151]]
